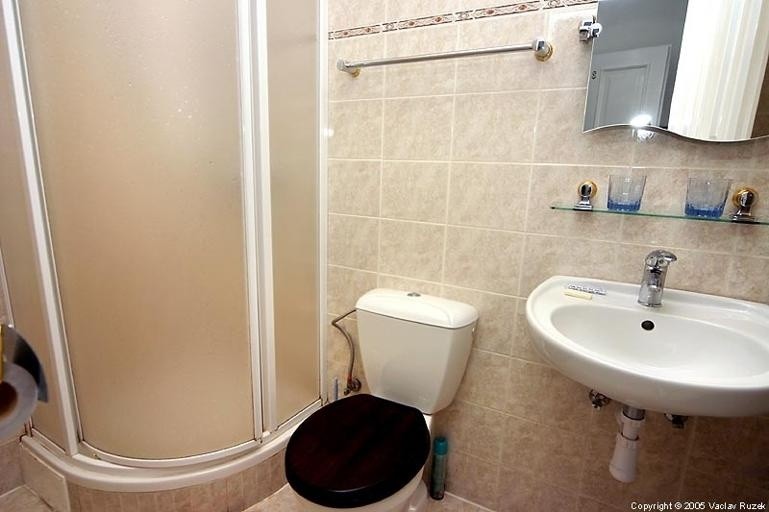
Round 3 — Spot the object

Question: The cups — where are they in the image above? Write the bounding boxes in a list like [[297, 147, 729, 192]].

[[687, 177, 730, 215], [607, 176, 646, 213]]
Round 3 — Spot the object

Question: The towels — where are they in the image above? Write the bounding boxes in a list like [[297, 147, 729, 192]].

[[0, 360, 52, 452]]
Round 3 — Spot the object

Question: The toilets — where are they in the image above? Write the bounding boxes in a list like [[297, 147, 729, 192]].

[[283, 287, 477, 511]]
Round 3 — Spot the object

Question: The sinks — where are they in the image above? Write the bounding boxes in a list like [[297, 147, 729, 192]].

[[523, 275, 769, 417]]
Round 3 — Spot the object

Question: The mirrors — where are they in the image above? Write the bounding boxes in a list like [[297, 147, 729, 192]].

[[583, 1, 769, 142]]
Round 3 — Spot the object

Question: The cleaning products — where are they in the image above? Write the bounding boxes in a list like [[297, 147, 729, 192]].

[[430, 436, 451, 502]]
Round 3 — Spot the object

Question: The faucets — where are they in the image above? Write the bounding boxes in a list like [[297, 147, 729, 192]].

[[637, 250, 676, 307]]
